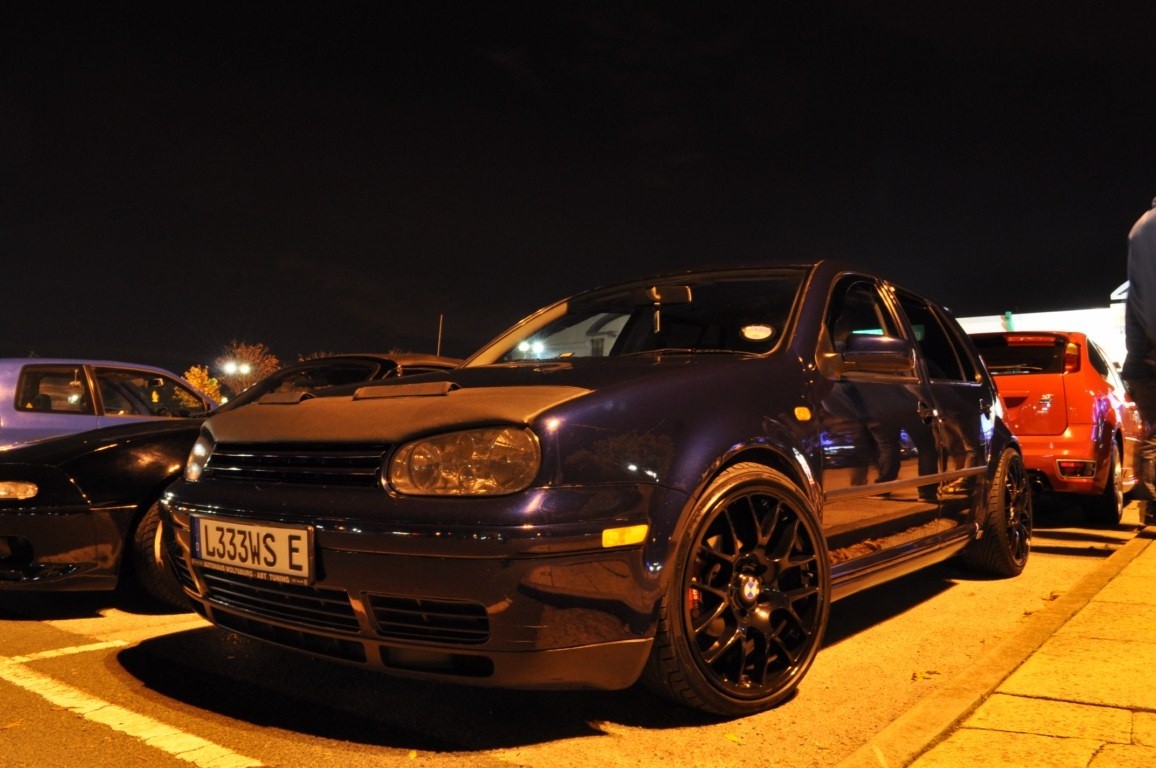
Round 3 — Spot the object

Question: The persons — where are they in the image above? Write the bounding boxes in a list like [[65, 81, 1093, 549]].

[[1124, 201, 1156, 423], [823, 380, 981, 502]]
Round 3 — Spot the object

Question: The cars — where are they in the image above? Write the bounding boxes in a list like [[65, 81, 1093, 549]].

[[159, 255, 1033, 719], [968, 333, 1149, 526], [0, 353, 465, 612], [0, 359, 220, 446]]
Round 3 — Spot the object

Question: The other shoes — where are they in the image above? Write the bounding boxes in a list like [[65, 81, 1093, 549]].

[[1137, 525, 1156, 539], [920, 493, 937, 501], [869, 492, 891, 499], [940, 479, 976, 493]]
